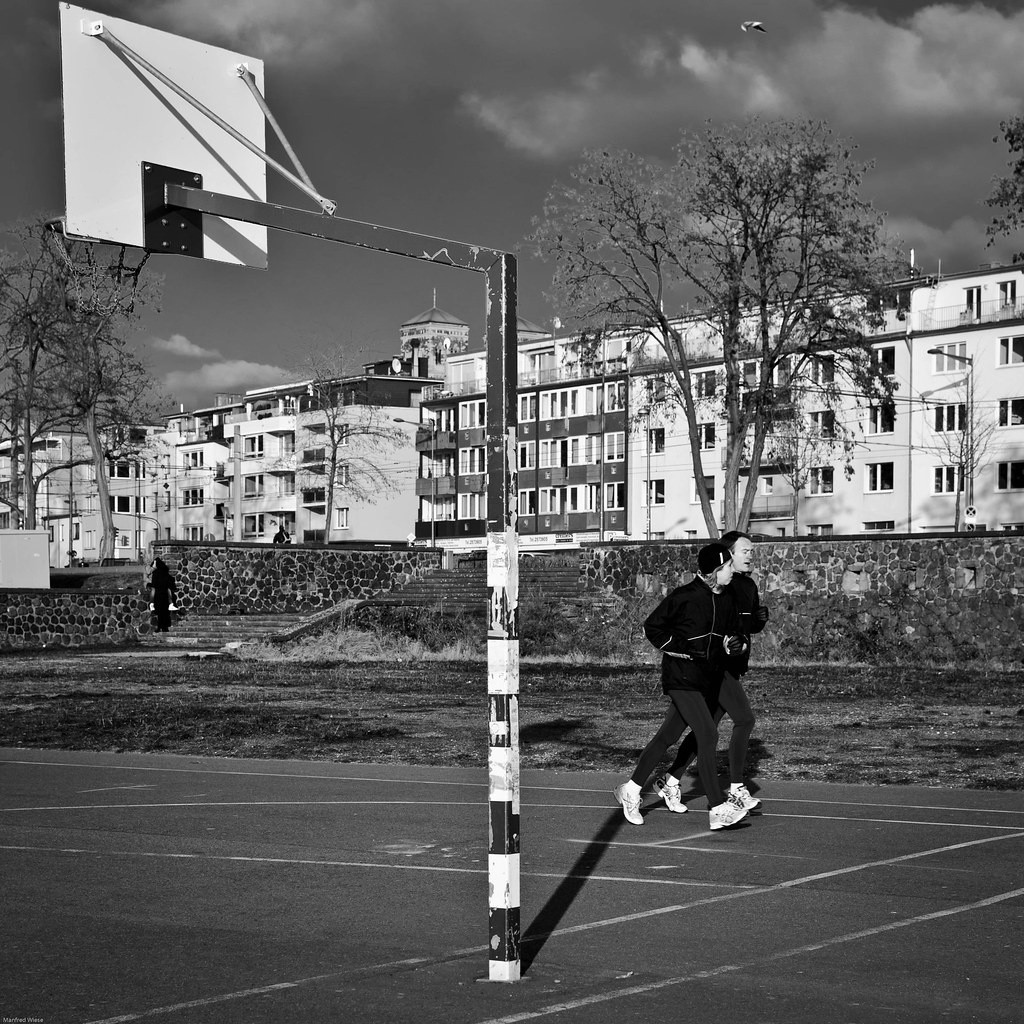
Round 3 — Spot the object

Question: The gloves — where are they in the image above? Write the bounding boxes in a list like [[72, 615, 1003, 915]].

[[727, 631, 744, 653], [756, 607, 769, 622], [689, 643, 706, 659]]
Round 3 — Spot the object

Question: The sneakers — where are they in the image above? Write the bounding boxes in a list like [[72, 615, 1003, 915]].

[[652, 775, 688, 814], [727, 785, 762, 810], [708, 802, 748, 830], [614, 783, 644, 825]]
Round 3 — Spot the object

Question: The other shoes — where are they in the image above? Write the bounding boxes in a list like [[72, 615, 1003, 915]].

[[150, 603, 155, 612], [162, 627, 169, 632], [168, 603, 178, 611]]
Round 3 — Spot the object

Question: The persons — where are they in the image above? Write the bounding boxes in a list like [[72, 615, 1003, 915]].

[[612, 538, 748, 831], [138, 552, 145, 565], [653, 526, 769, 815], [273, 525, 289, 543], [144, 558, 178, 633]]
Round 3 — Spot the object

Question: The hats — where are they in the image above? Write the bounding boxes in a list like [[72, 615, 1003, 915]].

[[698, 543, 731, 574]]
